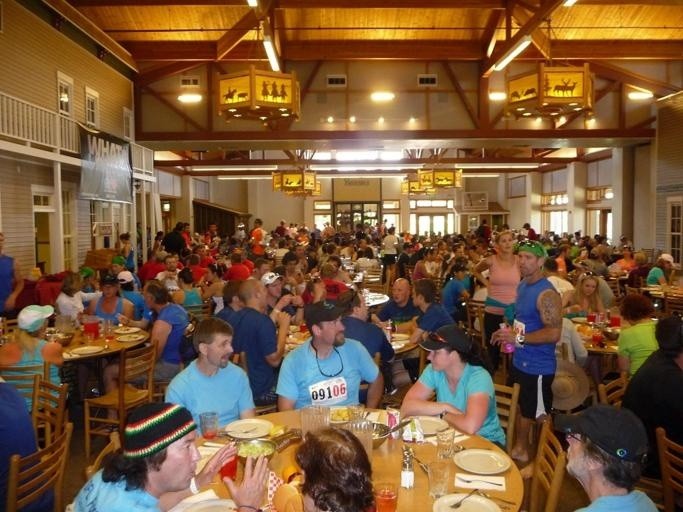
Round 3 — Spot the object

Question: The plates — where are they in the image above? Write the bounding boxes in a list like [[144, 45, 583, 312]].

[[328, 404, 350, 424], [431, 494, 502, 512], [70, 326, 146, 355], [401, 415, 449, 436], [640, 285, 662, 296], [572, 317, 586, 323], [390, 333, 410, 350], [454, 448, 510, 474], [184, 499, 239, 511], [224, 418, 274, 439]]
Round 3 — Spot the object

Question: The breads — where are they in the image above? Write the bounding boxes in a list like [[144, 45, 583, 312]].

[[272, 466, 304, 512]]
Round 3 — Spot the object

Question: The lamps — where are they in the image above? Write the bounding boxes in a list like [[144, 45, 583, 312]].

[[399, 179, 439, 194], [505, 19, 594, 118], [217, 20, 302, 124], [271, 170, 316, 192], [417, 161, 464, 190], [285, 180, 321, 197]]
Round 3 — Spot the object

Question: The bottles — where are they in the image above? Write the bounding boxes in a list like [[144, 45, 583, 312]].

[[385, 319, 393, 333], [400, 448, 415, 489], [502, 324, 515, 354], [343, 403, 373, 470]]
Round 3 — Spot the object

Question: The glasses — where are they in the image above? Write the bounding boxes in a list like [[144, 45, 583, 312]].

[[583, 271, 597, 277], [268, 272, 279, 279], [566, 432, 583, 444], [428, 330, 448, 345], [518, 240, 543, 253]]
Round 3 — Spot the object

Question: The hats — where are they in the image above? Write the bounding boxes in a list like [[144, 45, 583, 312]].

[[551, 360, 589, 411], [116, 270, 133, 284], [17, 304, 54, 333], [553, 405, 649, 464], [261, 272, 282, 287], [658, 253, 675, 268], [305, 301, 345, 321], [101, 274, 116, 284], [122, 403, 197, 459], [417, 324, 470, 352]]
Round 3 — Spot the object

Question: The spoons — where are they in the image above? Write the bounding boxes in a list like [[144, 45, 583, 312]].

[[401, 444, 428, 474], [476, 491, 515, 505], [218, 426, 255, 434]]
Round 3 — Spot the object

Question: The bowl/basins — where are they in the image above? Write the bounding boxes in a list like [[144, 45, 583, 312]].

[[339, 422, 392, 449], [45, 332, 73, 347], [235, 439, 277, 466], [601, 327, 619, 340]]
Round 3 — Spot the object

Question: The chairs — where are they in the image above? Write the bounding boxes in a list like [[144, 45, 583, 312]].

[[0, 236, 683, 512]]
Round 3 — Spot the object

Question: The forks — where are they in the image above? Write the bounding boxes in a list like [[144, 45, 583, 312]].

[[448, 488, 480, 509], [458, 475, 502, 487]]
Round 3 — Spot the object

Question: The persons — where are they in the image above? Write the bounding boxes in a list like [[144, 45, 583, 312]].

[[0, 217, 683, 512]]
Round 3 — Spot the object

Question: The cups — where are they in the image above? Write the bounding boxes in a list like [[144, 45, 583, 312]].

[[372, 482, 398, 512], [578, 309, 611, 346], [426, 461, 449, 497], [221, 456, 237, 482], [300, 324, 307, 332], [199, 412, 218, 439], [435, 426, 456, 458], [301, 405, 331, 443], [610, 316, 620, 327], [83, 316, 100, 339], [104, 319, 113, 341]]
